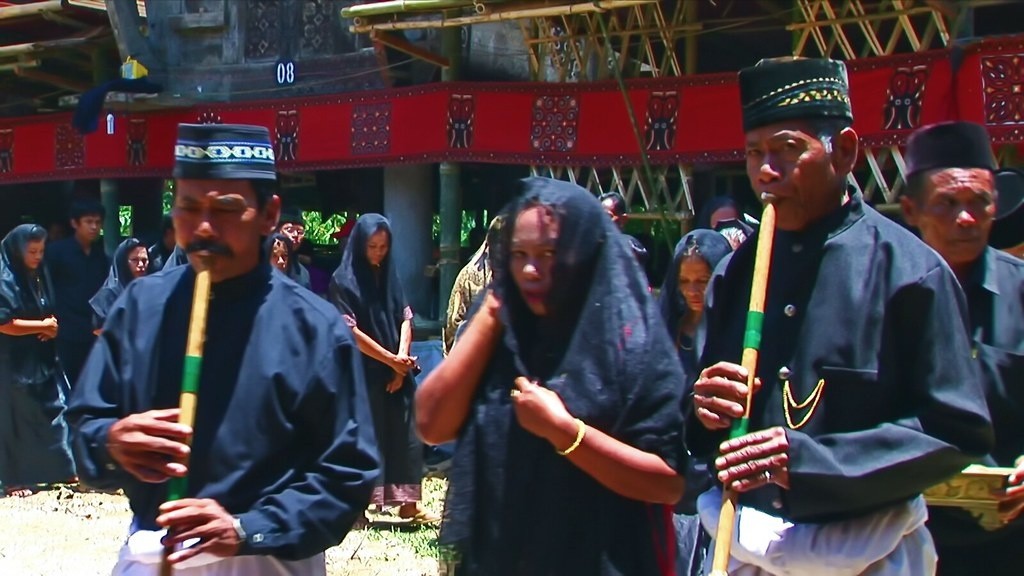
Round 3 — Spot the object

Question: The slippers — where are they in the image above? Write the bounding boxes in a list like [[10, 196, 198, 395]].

[[403, 512, 442, 526]]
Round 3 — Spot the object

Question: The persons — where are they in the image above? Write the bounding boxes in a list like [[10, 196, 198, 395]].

[[0, 55, 1024, 576]]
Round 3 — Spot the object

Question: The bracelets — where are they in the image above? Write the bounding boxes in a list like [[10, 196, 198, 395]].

[[555, 417, 587, 456]]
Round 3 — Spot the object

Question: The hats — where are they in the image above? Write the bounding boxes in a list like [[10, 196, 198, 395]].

[[985, 166, 1024, 249], [739, 57, 854, 134], [174, 120, 278, 181], [902, 119, 998, 179]]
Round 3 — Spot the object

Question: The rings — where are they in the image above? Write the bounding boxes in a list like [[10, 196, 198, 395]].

[[765, 470, 772, 485]]
